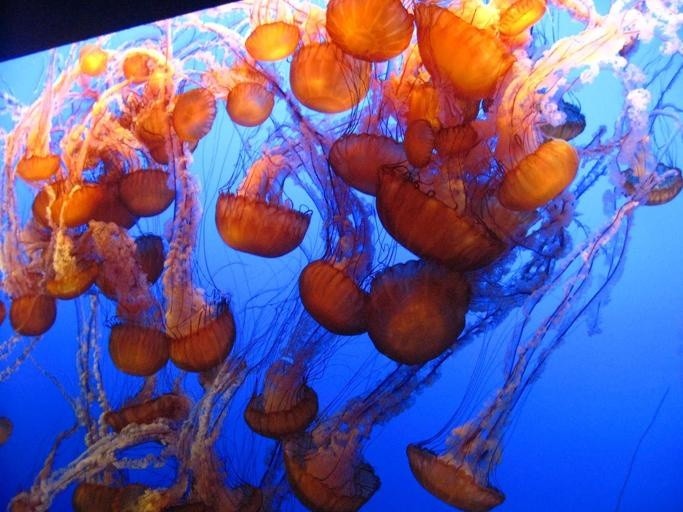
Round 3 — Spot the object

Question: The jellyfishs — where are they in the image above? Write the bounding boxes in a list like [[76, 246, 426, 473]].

[[0, 0, 682, 512]]
